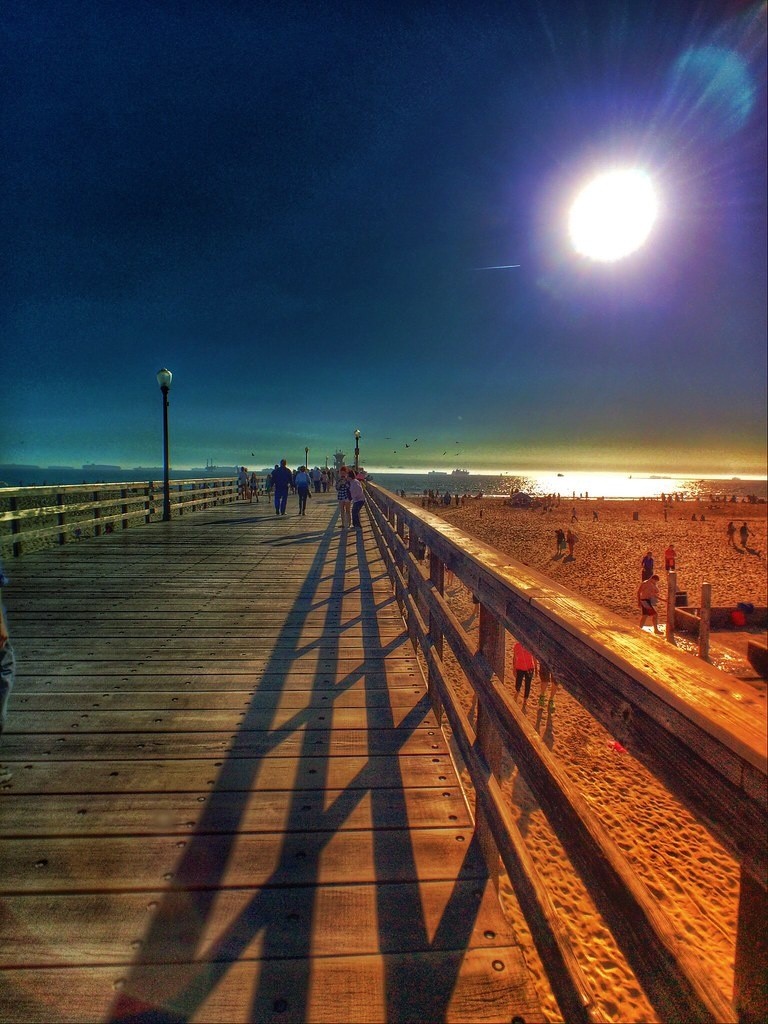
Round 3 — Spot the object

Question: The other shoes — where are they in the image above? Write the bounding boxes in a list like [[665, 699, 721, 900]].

[[347, 524, 351, 529], [269, 500, 271, 503], [281, 512, 288, 515], [0, 766, 12, 784], [257, 500, 259, 502], [302, 512, 306, 515], [298, 511, 302, 515], [350, 526, 361, 531], [249, 501, 252, 502], [276, 511, 280, 515], [339, 525, 346, 529]]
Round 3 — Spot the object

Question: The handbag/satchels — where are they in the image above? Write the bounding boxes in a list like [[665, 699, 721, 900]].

[[247, 491, 252, 500], [346, 487, 352, 501], [307, 486, 312, 499]]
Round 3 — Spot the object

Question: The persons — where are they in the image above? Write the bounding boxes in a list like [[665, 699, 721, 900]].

[[638, 545, 677, 635], [395, 487, 483, 519], [542, 493, 598, 558], [664, 506, 757, 549], [239, 458, 367, 532], [0, 571, 16, 783], [419, 538, 560, 709]]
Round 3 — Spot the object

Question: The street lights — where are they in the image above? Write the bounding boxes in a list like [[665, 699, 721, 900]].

[[325, 455, 329, 469], [304, 446, 310, 469], [156, 367, 173, 521], [353, 429, 361, 473]]
